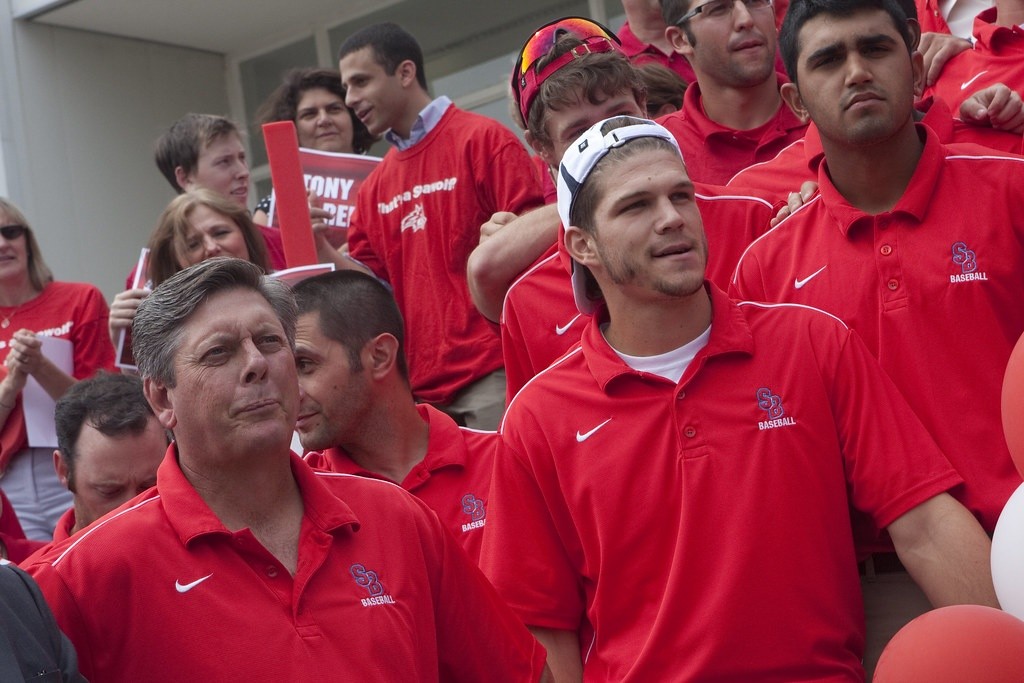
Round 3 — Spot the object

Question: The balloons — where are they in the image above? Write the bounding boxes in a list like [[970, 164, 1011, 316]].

[[990, 482, 1024, 621], [1001, 331, 1024, 481], [871, 605, 1024, 683]]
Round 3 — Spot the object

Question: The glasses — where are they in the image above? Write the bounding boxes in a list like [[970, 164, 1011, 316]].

[[0, 225, 25, 239], [675, 0, 775, 26], [513, 16, 622, 129]]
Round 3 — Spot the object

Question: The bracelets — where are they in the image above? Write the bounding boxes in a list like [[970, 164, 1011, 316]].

[[0, 402, 15, 410]]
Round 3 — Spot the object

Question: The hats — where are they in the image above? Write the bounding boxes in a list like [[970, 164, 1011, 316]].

[[557, 115, 688, 316], [510, 38, 629, 126]]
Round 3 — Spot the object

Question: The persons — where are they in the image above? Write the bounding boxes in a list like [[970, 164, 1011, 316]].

[[0, 0, 1024, 683]]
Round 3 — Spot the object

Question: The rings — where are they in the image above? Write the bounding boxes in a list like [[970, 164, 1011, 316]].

[[24, 356, 30, 363]]
